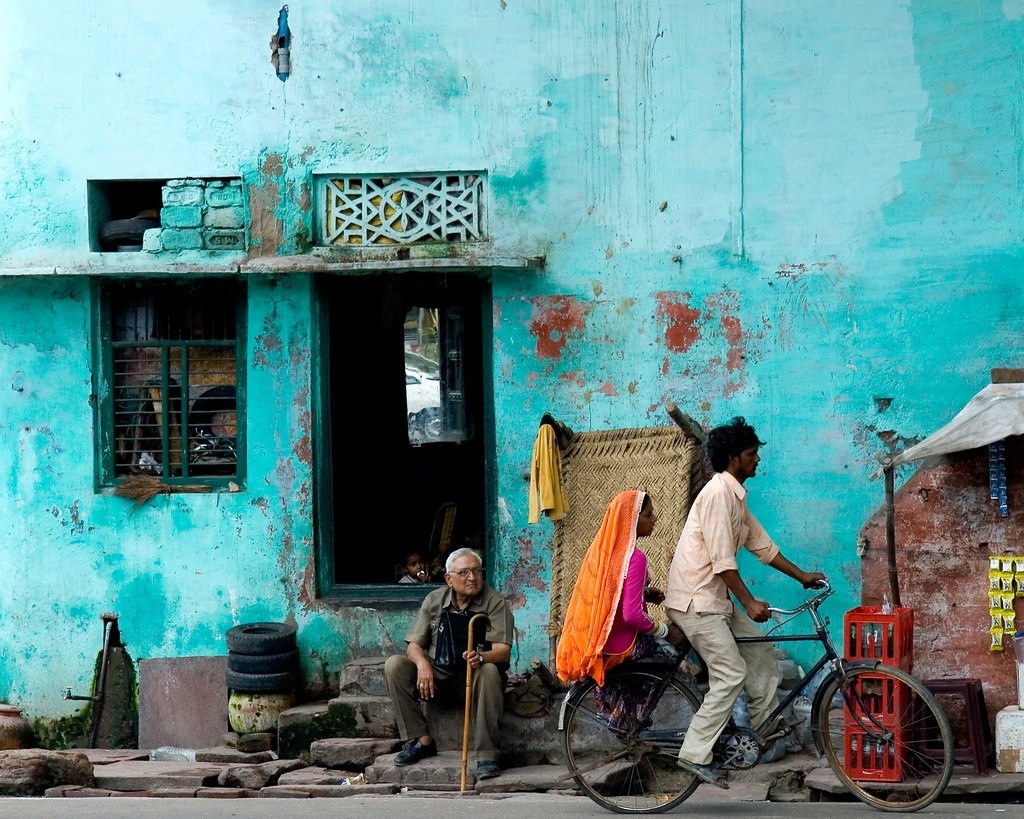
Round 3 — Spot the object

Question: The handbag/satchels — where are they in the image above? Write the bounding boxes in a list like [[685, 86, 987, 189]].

[[502, 675, 555, 719], [434, 610, 491, 670]]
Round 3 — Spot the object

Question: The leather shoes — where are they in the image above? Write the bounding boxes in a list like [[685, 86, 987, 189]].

[[476, 760, 500, 780], [393, 737, 437, 766]]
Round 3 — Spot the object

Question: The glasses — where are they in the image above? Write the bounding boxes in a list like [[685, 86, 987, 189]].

[[449, 566, 483, 576]]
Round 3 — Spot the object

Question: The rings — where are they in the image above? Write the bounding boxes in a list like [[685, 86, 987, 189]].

[[419, 682, 424, 687]]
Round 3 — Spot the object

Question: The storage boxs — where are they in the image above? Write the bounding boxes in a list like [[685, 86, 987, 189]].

[[843, 605, 913, 783]]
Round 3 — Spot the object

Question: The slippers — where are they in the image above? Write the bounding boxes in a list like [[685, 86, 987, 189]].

[[677, 759, 729, 789], [760, 716, 808, 755]]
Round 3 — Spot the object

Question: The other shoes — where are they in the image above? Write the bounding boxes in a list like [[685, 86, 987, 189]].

[[617, 717, 653, 740], [608, 718, 641, 747]]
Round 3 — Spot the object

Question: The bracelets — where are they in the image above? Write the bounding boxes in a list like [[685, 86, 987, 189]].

[[646, 622, 668, 640]]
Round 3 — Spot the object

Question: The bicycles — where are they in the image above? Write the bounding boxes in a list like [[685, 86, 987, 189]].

[[554, 579, 953, 813]]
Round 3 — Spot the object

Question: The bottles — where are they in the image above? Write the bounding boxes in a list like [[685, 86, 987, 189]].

[[849, 592, 895, 770]]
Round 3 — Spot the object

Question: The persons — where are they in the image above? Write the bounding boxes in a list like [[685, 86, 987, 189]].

[[666, 422, 830, 788], [557, 490, 686, 737], [398, 551, 430, 582], [385, 548, 516, 780]]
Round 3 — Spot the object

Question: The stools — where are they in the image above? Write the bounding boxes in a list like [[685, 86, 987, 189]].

[[914, 678, 996, 778]]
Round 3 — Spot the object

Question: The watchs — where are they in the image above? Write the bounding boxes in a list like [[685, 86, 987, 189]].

[[478, 653, 484, 665]]
